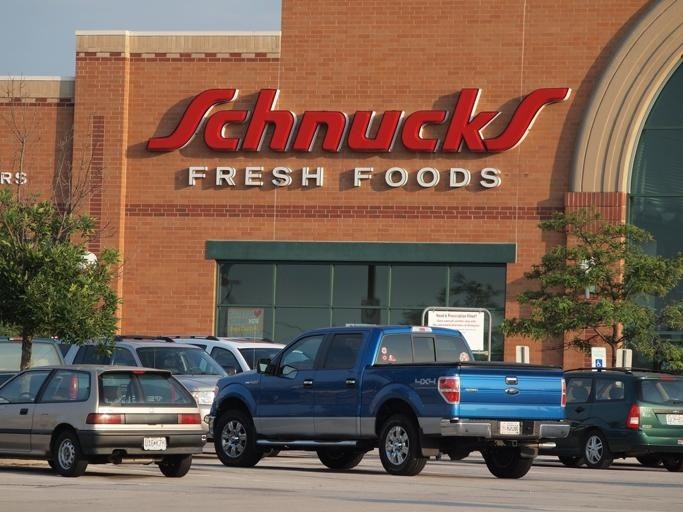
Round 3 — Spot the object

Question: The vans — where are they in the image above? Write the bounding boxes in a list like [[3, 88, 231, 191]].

[[0, 336, 66, 387], [556, 366, 683, 472]]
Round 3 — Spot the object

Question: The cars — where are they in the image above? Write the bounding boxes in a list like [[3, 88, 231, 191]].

[[0, 364, 208, 478]]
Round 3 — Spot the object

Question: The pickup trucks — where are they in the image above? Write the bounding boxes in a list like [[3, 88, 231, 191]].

[[203, 324, 571, 476]]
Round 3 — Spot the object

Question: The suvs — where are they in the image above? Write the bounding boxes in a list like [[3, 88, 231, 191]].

[[167, 335, 310, 458], [54, 334, 230, 441]]
